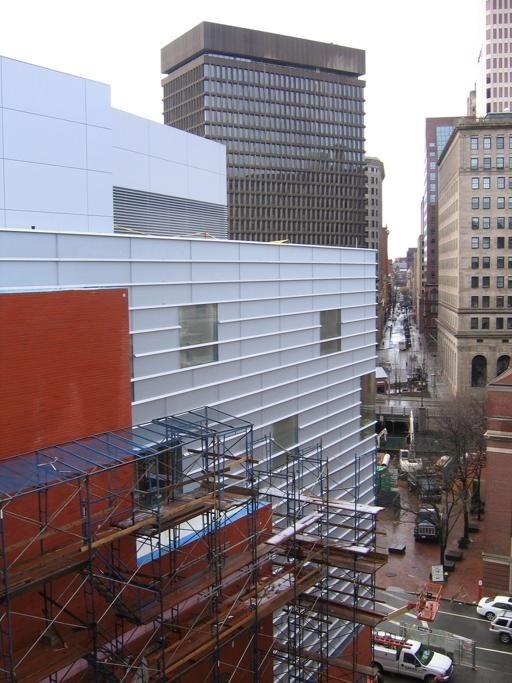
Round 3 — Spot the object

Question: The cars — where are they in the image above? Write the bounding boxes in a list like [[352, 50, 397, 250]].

[[476, 595, 512, 621], [488, 610, 511, 644]]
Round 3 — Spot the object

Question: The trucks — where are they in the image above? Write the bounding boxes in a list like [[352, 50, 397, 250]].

[[413, 504, 442, 541], [406, 471, 441, 501]]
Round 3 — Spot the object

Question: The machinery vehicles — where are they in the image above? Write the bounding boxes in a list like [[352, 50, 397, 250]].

[[398, 409, 424, 479]]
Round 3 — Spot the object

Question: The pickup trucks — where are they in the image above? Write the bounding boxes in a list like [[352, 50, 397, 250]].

[[371, 630, 454, 682]]
